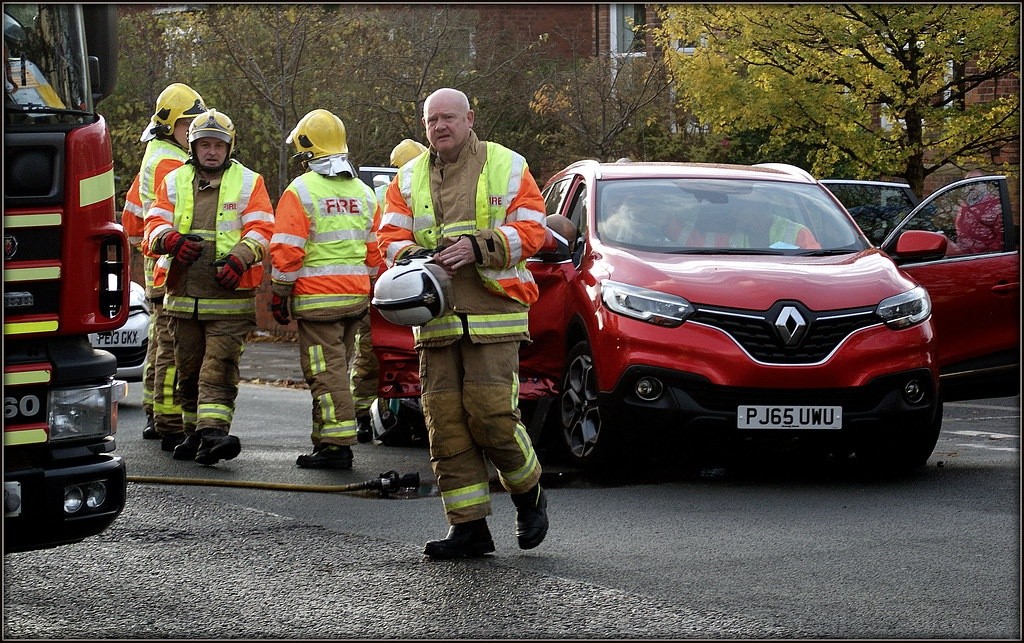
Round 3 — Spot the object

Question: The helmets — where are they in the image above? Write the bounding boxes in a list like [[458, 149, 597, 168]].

[[390, 138, 428, 168], [372, 257, 455, 326], [285, 108, 349, 164], [151, 83, 209, 135], [188, 108, 237, 159]]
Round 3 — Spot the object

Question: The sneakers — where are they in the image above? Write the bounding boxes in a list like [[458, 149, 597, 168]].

[[160, 432, 197, 460], [511, 483, 550, 549], [297, 445, 354, 469], [143, 411, 159, 439], [194, 432, 241, 465], [424, 517, 495, 560]]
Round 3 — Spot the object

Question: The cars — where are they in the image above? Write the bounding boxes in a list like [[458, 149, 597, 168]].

[[92, 264, 157, 385]]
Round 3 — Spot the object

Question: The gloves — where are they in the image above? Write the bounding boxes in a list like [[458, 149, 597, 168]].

[[272, 293, 291, 326], [164, 231, 204, 268], [213, 254, 244, 290]]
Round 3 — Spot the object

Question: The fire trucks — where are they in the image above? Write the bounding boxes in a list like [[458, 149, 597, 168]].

[[0, 0, 136, 557]]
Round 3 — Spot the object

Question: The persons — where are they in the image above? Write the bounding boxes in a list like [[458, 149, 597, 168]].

[[272, 109, 382, 468], [663, 193, 821, 248], [123, 122, 155, 437], [137, 82, 212, 450], [142, 109, 274, 466], [376, 90, 549, 559], [955, 171, 1008, 253], [350, 137, 428, 444]]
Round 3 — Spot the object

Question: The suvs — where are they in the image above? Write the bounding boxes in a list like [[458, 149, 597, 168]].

[[356, 155, 1021, 481]]
